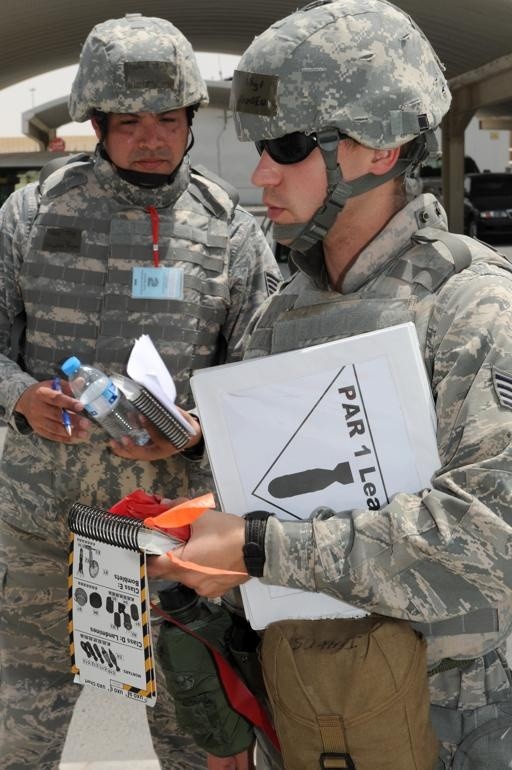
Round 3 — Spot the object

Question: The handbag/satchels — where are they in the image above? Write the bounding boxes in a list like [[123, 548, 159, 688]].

[[262, 613, 440, 769]]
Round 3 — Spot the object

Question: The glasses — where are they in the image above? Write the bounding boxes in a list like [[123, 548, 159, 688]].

[[254, 126, 351, 166]]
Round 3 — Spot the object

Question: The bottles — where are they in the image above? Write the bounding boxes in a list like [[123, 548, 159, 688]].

[[59, 356, 151, 450]]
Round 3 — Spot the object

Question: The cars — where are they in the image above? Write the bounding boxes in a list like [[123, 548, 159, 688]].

[[415, 154, 512, 244]]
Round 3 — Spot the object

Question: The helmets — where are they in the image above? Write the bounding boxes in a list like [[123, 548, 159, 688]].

[[68, 14, 211, 122], [231, 0, 453, 152]]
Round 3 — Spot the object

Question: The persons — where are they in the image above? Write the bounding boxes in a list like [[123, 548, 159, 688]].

[[143, 2, 511, 770], [0, 0, 288, 770]]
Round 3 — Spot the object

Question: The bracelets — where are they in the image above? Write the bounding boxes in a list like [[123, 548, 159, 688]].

[[241, 510, 276, 581]]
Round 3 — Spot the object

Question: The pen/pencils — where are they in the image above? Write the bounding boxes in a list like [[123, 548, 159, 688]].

[[53, 377, 72, 436]]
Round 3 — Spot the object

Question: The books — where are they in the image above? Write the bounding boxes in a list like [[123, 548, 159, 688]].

[[187, 319, 445, 633], [61, 500, 187, 709], [106, 334, 196, 449]]
